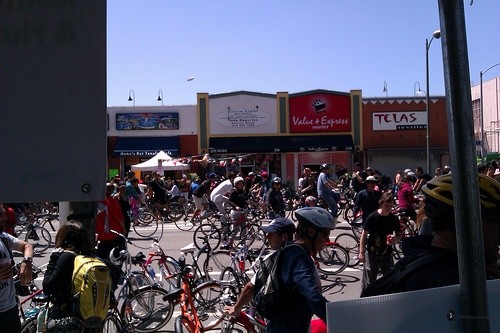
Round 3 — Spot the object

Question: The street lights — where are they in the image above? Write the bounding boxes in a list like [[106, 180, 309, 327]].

[[425, 30, 441, 175]]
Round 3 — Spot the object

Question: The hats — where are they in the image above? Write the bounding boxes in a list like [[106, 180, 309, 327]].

[[259, 216, 295, 234]]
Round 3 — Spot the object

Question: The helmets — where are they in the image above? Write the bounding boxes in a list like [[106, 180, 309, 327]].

[[421, 171, 500, 212], [295, 207, 337, 230], [208, 163, 416, 191]]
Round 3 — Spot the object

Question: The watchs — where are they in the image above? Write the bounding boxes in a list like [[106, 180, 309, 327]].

[[22, 256, 33, 264]]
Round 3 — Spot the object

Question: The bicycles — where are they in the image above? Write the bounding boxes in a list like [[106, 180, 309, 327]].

[[0, 174, 425, 333]]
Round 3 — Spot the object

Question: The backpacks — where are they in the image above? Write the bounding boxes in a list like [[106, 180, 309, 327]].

[[61, 248, 111, 330], [251, 243, 309, 324]]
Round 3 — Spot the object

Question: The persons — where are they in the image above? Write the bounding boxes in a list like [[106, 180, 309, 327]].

[[265, 206, 336, 333], [41, 219, 101, 333], [105, 159, 500, 227], [0, 202, 33, 333], [356, 192, 403, 299], [96, 193, 127, 293], [229, 175, 250, 252], [391, 172, 500, 293], [223, 217, 296, 326]]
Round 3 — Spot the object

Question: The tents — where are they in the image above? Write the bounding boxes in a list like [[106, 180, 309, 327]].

[[131, 149, 191, 182]]
